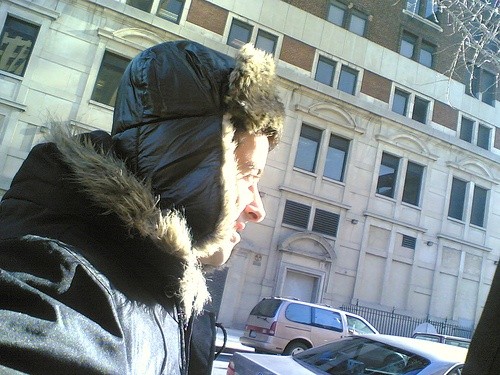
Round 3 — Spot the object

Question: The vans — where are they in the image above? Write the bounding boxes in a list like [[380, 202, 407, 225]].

[[240, 294, 381, 358]]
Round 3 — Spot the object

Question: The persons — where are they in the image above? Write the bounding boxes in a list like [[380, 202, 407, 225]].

[[0, 39, 285, 375]]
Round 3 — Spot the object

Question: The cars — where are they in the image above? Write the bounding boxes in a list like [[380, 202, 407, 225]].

[[411, 331, 472, 348], [227, 334, 469, 375]]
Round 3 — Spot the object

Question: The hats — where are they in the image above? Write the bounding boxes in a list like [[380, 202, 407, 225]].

[[109, 39, 283, 256]]
